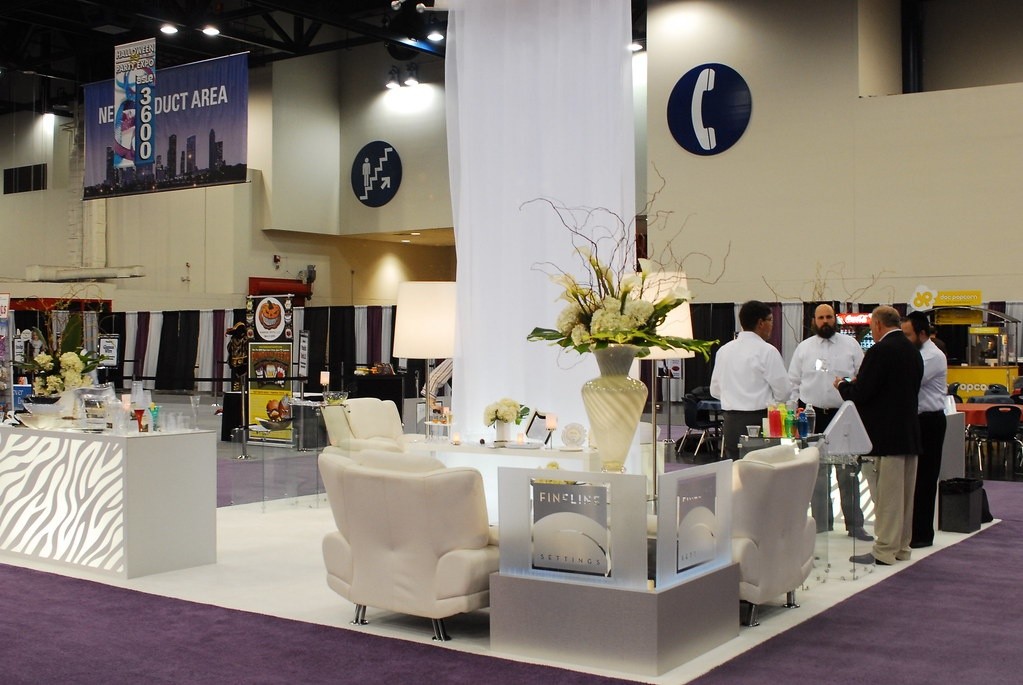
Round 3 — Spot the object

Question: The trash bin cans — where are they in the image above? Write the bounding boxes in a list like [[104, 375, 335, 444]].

[[221, 391, 249, 440], [938, 478, 983, 534], [290, 404, 329, 450]]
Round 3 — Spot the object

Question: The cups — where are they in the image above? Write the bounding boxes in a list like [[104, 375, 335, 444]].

[[108, 379, 201, 434], [763, 418, 770, 437]]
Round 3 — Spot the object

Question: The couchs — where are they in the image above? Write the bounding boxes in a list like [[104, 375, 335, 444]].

[[317, 449, 498, 642], [646, 445, 820, 627], [322, 398, 407, 448]]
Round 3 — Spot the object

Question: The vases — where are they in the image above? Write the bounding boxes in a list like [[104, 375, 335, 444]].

[[581, 344, 648, 473], [495, 418, 511, 441], [22, 394, 67, 414]]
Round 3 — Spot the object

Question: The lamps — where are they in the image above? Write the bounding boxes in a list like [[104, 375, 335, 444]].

[[633, 270, 694, 444], [387, 63, 420, 87]]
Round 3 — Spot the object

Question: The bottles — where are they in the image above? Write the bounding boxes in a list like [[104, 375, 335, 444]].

[[432, 409, 439, 424], [440, 409, 446, 424], [767, 397, 816, 438]]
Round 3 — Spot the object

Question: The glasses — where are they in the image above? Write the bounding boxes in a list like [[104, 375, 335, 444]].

[[759, 317, 773, 322]]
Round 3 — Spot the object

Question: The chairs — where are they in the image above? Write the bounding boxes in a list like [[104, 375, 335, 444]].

[[677, 388, 725, 457], [949, 382, 1023, 477]]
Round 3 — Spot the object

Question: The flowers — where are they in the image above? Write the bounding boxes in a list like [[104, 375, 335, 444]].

[[509, 162, 731, 362], [483, 397, 529, 425], [12, 283, 112, 399]]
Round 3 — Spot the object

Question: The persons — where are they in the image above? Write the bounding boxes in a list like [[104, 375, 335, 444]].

[[710, 301, 793, 462], [420, 359, 452, 415], [30, 329, 44, 358], [903, 313, 946, 550], [788, 305, 877, 541], [834, 306, 919, 566]]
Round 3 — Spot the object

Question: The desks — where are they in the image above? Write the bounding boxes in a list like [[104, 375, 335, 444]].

[[955, 404, 1023, 467], [0, 423, 217, 578]]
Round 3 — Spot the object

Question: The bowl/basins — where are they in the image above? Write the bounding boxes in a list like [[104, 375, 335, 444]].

[[322, 391, 348, 404], [13, 396, 65, 429], [746, 426, 761, 437]]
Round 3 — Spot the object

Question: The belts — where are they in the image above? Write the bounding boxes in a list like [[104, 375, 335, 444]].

[[799, 399, 840, 415]]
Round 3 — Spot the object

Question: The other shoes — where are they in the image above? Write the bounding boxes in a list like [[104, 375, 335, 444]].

[[849, 551, 890, 565], [848, 529, 873, 541]]
